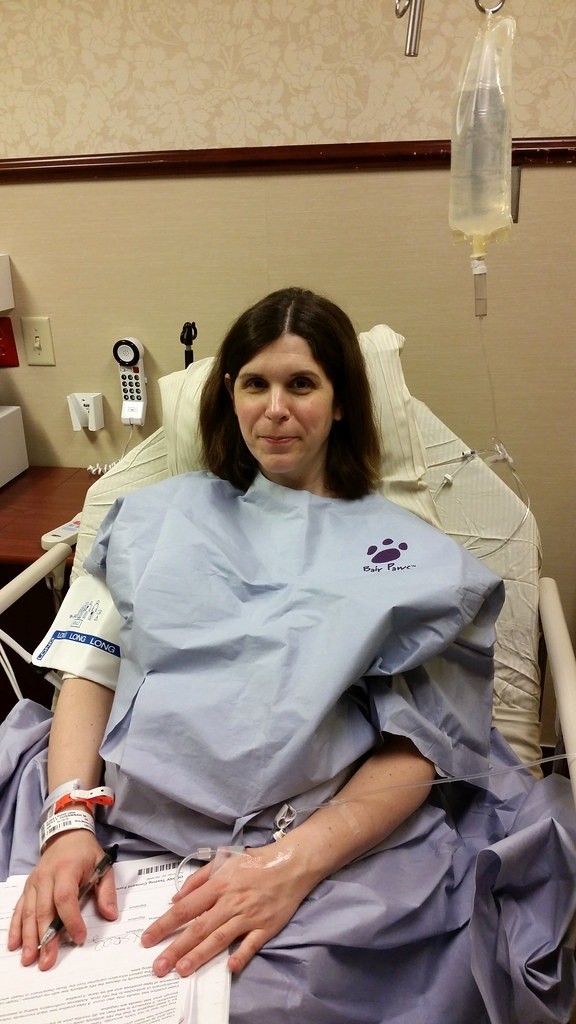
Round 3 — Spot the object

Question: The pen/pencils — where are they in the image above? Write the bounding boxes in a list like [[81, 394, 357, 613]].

[[36, 843, 119, 950]]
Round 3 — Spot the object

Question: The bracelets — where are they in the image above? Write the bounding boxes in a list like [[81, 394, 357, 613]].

[[35, 780, 114, 854]]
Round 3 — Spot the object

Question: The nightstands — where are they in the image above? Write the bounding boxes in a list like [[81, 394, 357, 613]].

[[0, 466, 103, 721]]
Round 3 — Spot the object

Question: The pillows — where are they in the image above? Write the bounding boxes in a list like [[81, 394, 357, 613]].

[[155, 322, 441, 531]]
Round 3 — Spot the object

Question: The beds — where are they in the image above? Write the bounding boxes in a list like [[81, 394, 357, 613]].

[[0, 398, 576, 1024]]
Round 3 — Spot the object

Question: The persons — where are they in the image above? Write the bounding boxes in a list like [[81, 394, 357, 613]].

[[6, 287, 505, 977]]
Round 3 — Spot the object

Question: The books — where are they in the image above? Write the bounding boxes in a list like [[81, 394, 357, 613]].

[[0, 852, 231, 1024]]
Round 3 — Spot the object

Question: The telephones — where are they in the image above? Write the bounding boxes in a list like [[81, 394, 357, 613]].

[[112, 336, 145, 426]]
